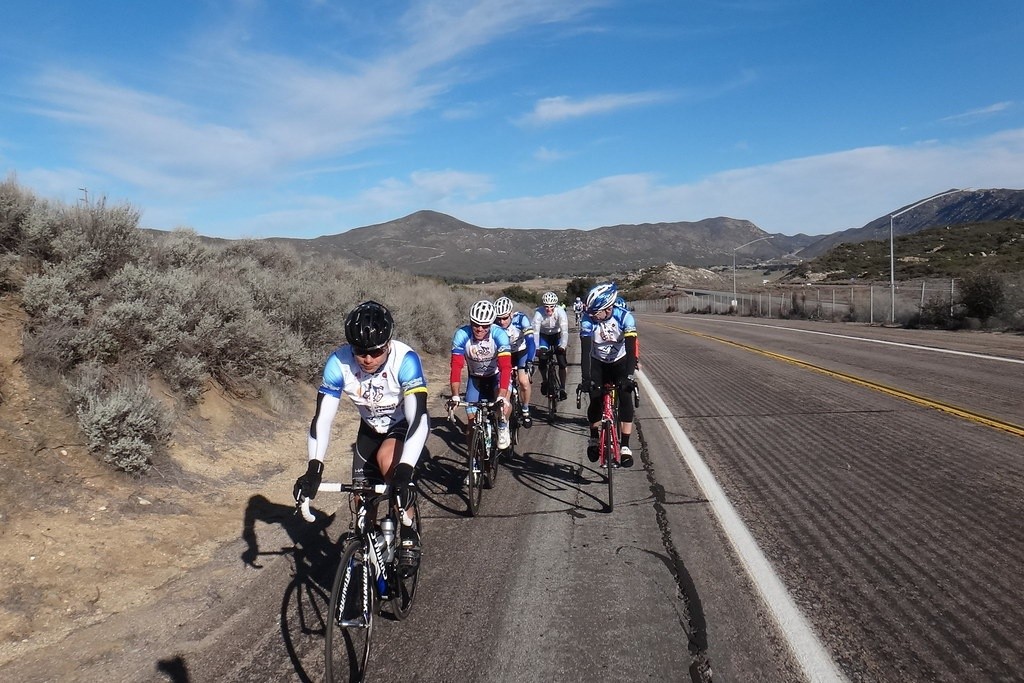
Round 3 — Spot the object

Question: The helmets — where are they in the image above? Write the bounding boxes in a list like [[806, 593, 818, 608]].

[[576, 297, 581, 301], [542, 292, 559, 304], [494, 297, 513, 318], [614, 297, 627, 310], [585, 283, 617, 312], [469, 299, 497, 326], [345, 301, 394, 350]]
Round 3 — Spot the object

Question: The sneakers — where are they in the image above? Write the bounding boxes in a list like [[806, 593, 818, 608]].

[[497, 420, 510, 449], [587, 437, 599, 463], [466, 459, 479, 485], [396, 531, 421, 579], [345, 566, 363, 619], [620, 446, 633, 468]]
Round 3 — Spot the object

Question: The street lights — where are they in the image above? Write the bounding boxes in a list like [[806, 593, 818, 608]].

[[733, 235, 776, 316], [890, 185, 975, 325]]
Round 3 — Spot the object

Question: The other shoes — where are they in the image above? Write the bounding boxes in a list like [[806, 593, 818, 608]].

[[541, 384, 547, 395], [560, 390, 567, 400], [522, 408, 532, 429]]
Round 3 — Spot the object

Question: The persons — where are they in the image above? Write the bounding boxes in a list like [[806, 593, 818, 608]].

[[444, 300, 512, 449], [294, 300, 430, 625], [580, 283, 639, 468], [559, 301, 566, 311], [493, 296, 536, 429], [573, 297, 583, 326], [531, 292, 568, 401]]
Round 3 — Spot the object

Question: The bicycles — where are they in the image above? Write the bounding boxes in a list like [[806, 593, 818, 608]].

[[507, 365, 535, 460], [444, 378, 509, 519], [294, 479, 424, 683], [533, 345, 567, 427], [575, 376, 641, 512], [574, 311, 586, 330]]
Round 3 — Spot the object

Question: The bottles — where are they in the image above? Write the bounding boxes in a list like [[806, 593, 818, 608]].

[[482, 420, 492, 461], [370, 519, 389, 564], [380, 513, 396, 563]]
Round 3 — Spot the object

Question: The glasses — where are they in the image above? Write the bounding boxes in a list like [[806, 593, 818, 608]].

[[352, 347, 388, 358], [471, 322, 490, 329], [592, 311, 602, 317], [544, 305, 555, 310], [497, 315, 510, 321]]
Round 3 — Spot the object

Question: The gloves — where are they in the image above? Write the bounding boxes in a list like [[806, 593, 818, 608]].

[[389, 463, 417, 511], [525, 361, 534, 376], [293, 459, 324, 505]]
